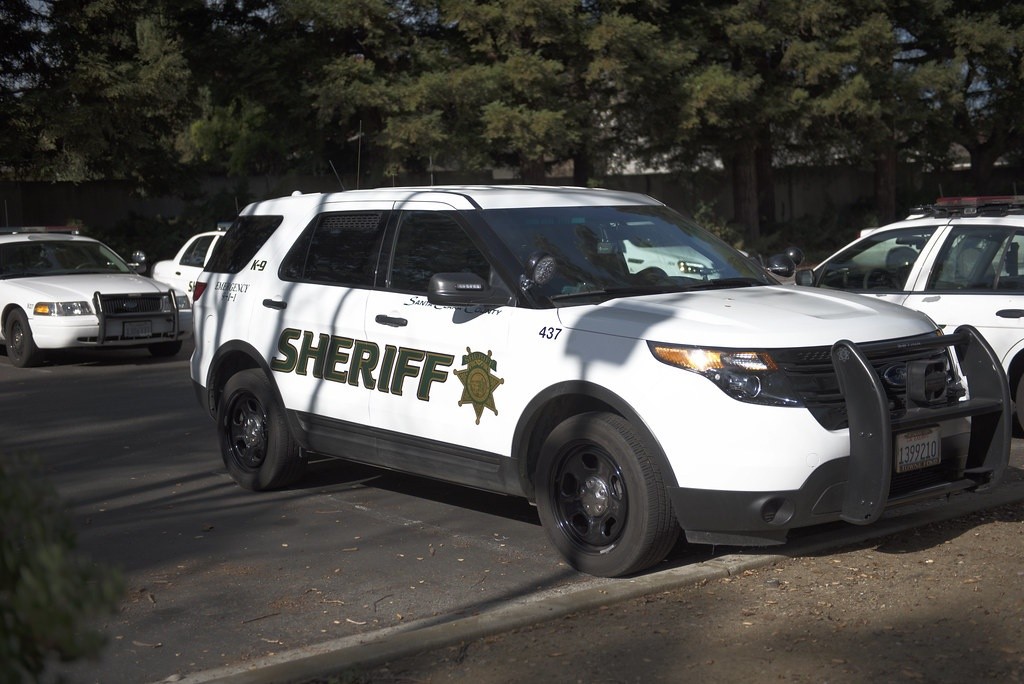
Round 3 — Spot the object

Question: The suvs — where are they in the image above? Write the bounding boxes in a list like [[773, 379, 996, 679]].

[[188, 183, 1012, 579]]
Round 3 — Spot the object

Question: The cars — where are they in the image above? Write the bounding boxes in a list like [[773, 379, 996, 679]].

[[152, 222, 233, 309], [794, 194, 1024, 440], [0, 226, 193, 368], [608, 221, 748, 282]]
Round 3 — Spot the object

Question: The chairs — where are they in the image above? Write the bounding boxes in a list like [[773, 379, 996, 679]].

[[957, 248, 997, 290]]
